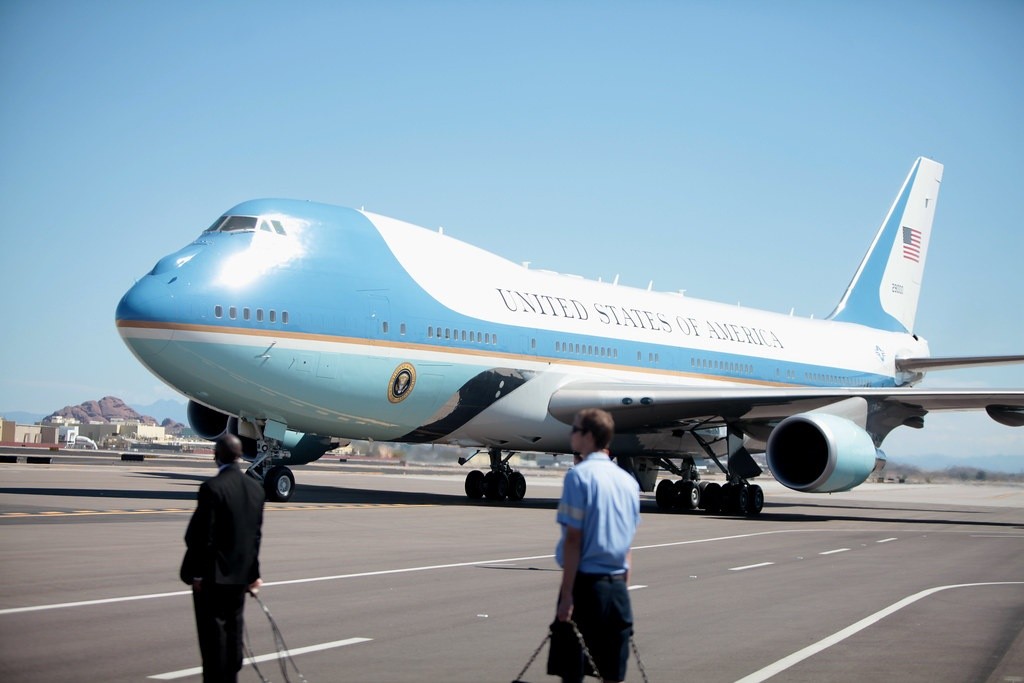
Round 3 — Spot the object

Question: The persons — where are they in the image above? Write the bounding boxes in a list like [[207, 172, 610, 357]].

[[547, 407, 640, 683], [180, 433, 265, 683]]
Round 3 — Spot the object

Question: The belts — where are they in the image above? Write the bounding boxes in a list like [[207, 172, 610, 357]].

[[577, 574, 625, 580]]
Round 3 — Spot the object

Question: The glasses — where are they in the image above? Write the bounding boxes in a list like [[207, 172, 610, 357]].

[[572, 425, 587, 433]]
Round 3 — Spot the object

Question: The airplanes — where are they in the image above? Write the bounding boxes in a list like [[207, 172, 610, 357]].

[[114, 155, 1024, 517]]
